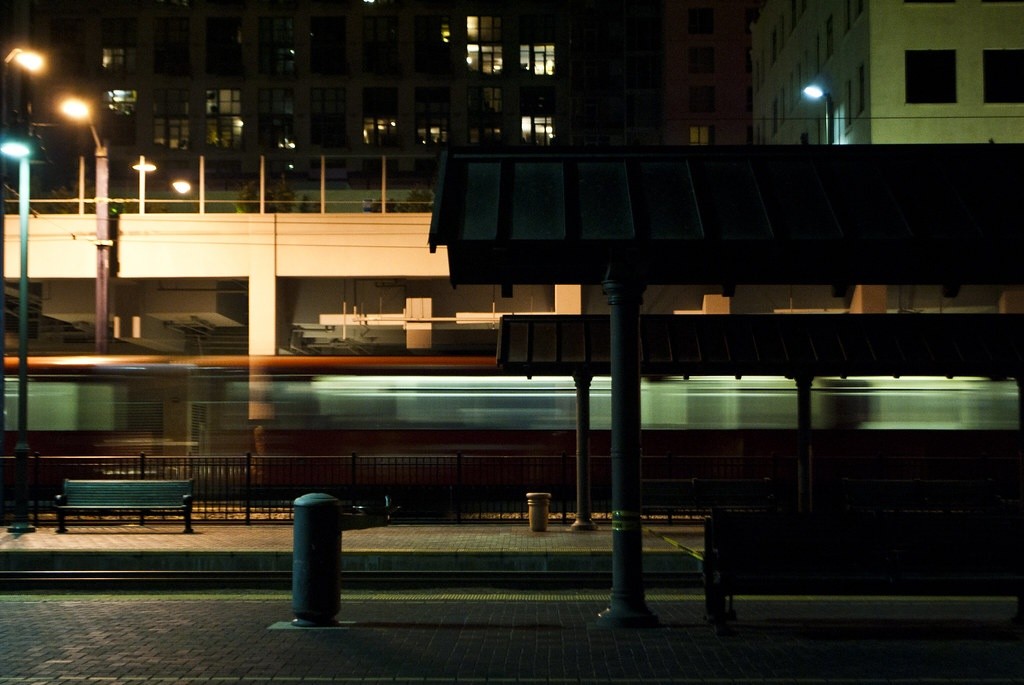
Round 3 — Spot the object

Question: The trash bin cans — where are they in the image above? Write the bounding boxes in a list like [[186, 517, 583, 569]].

[[290, 493, 342, 627]]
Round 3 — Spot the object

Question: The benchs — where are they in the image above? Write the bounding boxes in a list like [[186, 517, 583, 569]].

[[701, 508, 1024, 633], [639, 476, 774, 525], [842, 477, 993, 509], [51, 479, 194, 535]]
